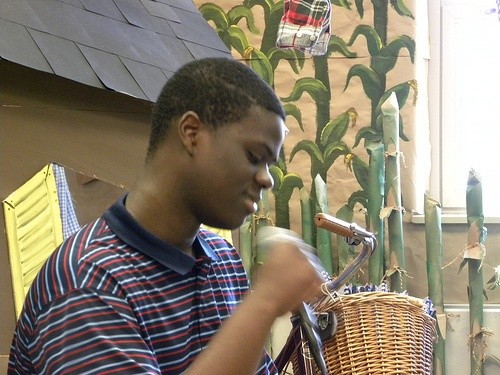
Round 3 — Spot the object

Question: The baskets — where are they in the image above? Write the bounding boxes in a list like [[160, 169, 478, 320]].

[[290, 290, 441, 375]]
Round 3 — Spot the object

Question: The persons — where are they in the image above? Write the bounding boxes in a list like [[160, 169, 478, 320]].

[[6, 57, 327, 374]]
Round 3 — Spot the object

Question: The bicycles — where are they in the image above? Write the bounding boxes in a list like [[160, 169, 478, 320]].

[[267, 213, 376, 375]]
